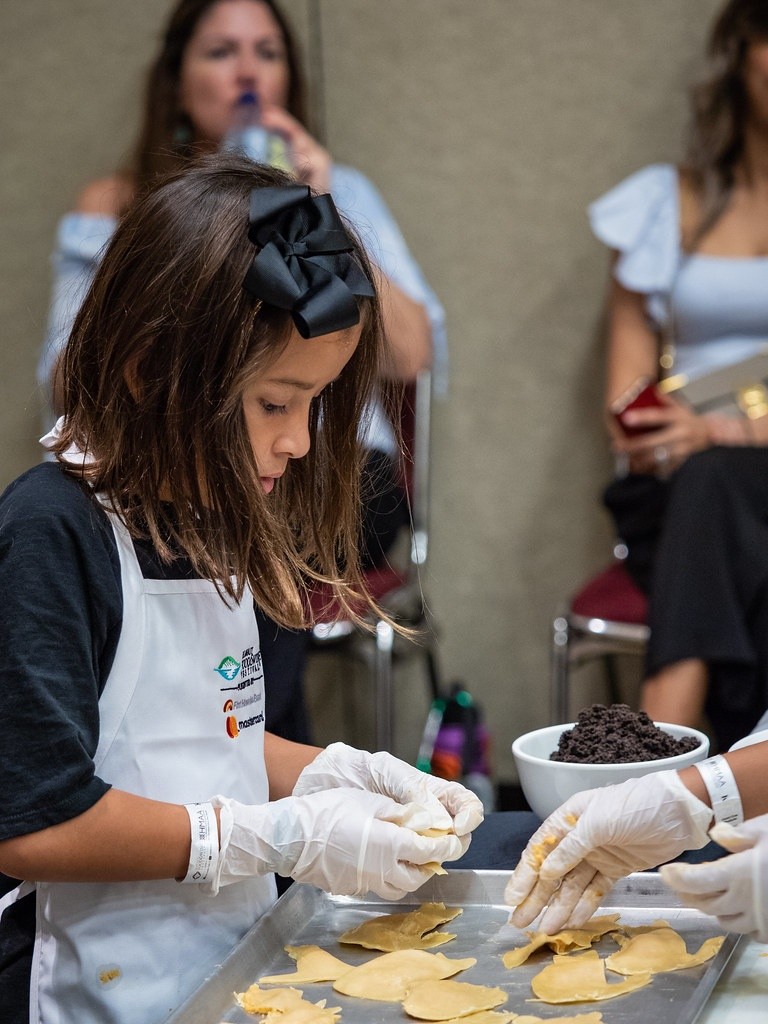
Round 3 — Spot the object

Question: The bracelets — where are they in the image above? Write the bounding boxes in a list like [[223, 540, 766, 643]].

[[691, 754, 744, 828], [176, 801, 218, 885]]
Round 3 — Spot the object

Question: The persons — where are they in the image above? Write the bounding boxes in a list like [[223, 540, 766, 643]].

[[583, 0, 768, 728], [37, 1, 454, 574], [504, 710, 768, 941], [0, 155, 486, 1024]]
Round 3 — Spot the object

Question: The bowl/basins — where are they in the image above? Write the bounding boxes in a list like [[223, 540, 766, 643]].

[[511, 721, 710, 823]]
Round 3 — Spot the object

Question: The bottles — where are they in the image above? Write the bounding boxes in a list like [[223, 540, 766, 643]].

[[219, 92, 269, 166]]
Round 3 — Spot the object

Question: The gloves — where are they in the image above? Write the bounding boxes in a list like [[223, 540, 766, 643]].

[[504, 769, 719, 936], [289, 742, 484, 861], [659, 814, 768, 948], [199, 796, 462, 902]]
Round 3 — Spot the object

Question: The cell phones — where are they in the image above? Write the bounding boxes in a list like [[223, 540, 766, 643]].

[[607, 374, 664, 442]]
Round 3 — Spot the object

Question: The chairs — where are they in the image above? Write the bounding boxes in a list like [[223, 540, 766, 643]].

[[551, 611, 649, 724], [300, 371, 438, 751]]
